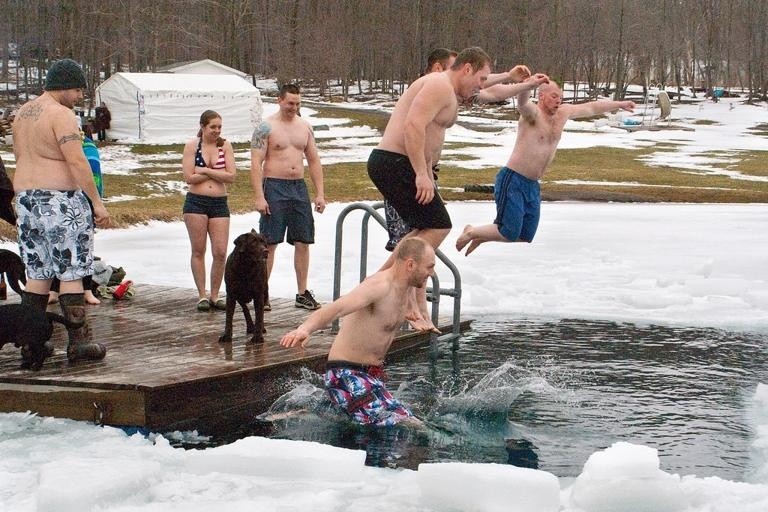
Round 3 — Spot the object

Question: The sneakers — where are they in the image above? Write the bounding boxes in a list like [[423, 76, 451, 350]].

[[294, 289, 322, 310], [111, 279, 133, 300], [263, 296, 272, 311]]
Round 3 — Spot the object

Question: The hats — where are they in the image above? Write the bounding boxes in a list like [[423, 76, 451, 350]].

[[39, 57, 89, 92]]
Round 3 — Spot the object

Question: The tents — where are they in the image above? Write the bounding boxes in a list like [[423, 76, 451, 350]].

[[89, 72, 265, 146]]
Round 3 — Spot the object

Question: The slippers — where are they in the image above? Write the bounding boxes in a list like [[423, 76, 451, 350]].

[[209, 295, 227, 310], [197, 297, 211, 311]]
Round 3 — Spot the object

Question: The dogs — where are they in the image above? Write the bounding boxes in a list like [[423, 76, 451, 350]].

[[218, 228, 270, 344], [1, 304, 84, 372]]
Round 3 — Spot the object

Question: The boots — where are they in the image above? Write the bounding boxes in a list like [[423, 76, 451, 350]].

[[58, 291, 108, 362], [18, 290, 56, 364]]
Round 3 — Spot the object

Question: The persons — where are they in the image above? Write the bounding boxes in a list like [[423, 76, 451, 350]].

[[384, 47, 552, 333], [181, 111, 239, 312], [96, 102, 112, 142], [46, 124, 104, 306], [11, 57, 115, 370], [454, 76, 638, 256], [269, 235, 435, 442], [74, 112, 82, 131], [249, 84, 328, 312], [364, 46, 493, 337]]
